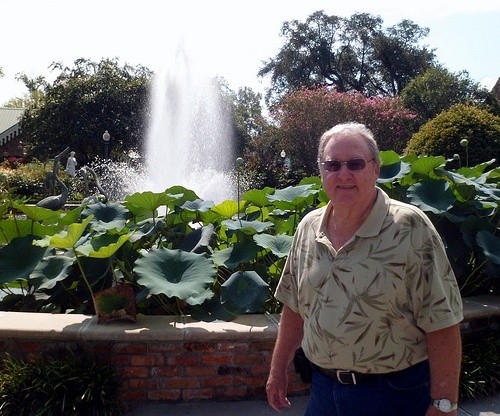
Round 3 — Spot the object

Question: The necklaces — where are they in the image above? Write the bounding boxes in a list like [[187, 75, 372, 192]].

[[332, 206, 371, 249]]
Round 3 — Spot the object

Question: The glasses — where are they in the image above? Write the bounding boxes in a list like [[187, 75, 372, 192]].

[[320, 158, 374, 171]]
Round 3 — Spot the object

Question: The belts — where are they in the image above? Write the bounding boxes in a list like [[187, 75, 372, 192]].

[[319, 364, 412, 384]]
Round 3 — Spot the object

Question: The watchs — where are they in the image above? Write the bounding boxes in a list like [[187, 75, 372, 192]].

[[430, 396, 458, 414]]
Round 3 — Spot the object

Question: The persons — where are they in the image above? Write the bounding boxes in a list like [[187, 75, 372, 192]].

[[65, 151, 78, 178], [264, 120, 468, 416]]
